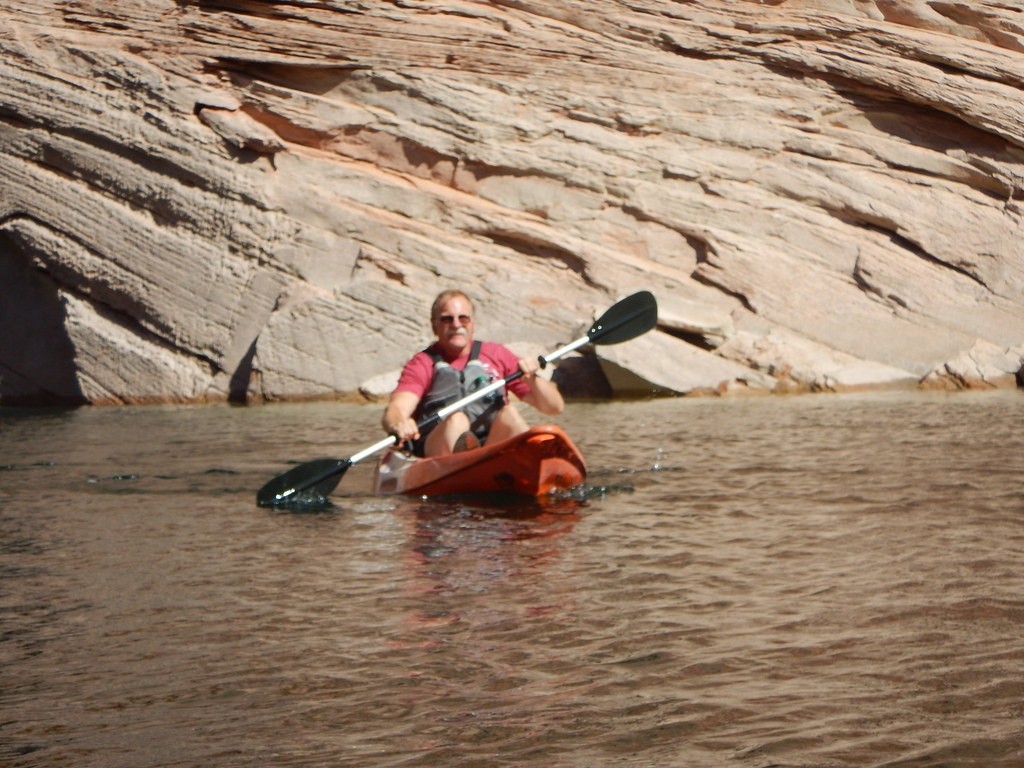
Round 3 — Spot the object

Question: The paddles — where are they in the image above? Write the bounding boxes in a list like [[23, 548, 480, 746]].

[[255, 290, 658, 510]]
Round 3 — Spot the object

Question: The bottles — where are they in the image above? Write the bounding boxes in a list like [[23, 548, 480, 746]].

[[482, 363, 499, 397], [463, 359, 487, 390], [434, 361, 454, 376]]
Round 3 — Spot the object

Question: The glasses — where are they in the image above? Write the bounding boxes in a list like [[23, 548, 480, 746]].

[[439, 315, 472, 325]]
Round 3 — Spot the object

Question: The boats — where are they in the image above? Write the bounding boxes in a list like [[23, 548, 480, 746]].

[[373, 425, 587, 496]]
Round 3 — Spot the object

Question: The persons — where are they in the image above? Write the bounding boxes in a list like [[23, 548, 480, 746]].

[[382, 289, 563, 457]]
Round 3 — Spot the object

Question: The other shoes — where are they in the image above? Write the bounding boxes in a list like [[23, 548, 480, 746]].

[[453, 431, 481, 452]]
